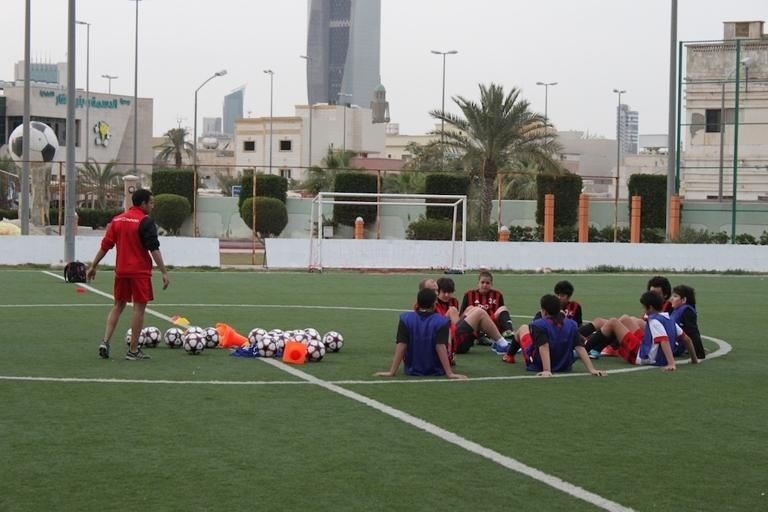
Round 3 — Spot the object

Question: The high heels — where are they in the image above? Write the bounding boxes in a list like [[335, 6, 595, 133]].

[[283, 340, 307, 365], [217, 324, 247, 348]]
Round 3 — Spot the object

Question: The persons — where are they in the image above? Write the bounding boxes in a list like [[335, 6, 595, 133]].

[[104, 193, 112, 199], [7, 180, 18, 209], [84, 188, 170, 360]]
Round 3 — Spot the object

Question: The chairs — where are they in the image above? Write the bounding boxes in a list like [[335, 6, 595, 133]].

[[98, 342, 109, 358], [588, 345, 618, 359], [476, 332, 521, 363], [125, 348, 150, 360]]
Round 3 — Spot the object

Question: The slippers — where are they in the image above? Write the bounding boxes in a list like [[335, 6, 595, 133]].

[[64, 262, 86, 283]]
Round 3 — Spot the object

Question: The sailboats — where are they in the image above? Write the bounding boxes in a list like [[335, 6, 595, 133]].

[[248, 328, 344, 361], [127, 325, 220, 355]]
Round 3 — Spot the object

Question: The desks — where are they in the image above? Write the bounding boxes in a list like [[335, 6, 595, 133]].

[[102, 73, 120, 94], [300, 55, 315, 171], [192, 69, 227, 171], [613, 88, 627, 195], [536, 80, 559, 153], [76, 21, 93, 165], [720, 56, 753, 202], [431, 49, 459, 162], [263, 69, 275, 171]]
